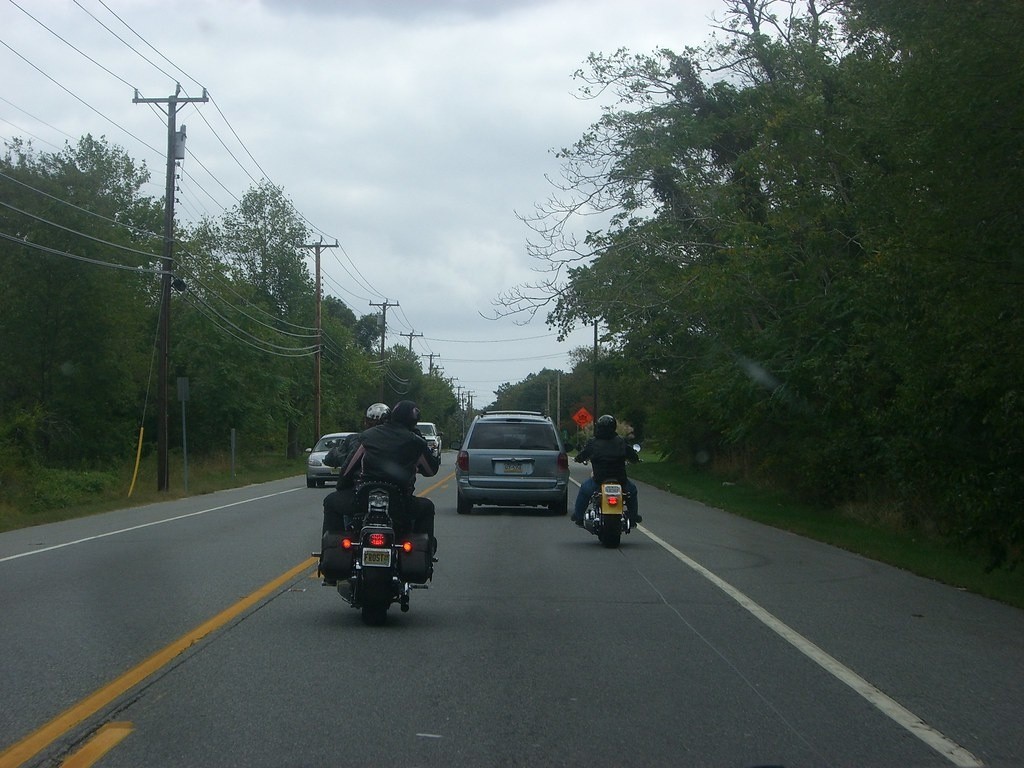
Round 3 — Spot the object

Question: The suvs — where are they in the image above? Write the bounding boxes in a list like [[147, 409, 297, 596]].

[[417, 422, 443, 465], [453, 411, 575, 516]]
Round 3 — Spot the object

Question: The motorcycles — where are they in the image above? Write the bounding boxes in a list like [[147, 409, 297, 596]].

[[311, 513, 439, 627], [574, 443, 641, 547]]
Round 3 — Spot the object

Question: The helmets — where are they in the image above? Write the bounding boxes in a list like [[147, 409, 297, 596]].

[[391, 400, 421, 420], [365, 403, 390, 420], [597, 414, 617, 433]]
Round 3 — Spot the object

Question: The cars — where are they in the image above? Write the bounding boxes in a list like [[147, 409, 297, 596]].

[[305, 432, 361, 488]]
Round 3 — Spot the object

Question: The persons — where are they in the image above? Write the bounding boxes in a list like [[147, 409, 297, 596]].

[[322, 400, 439, 589], [571, 415, 638, 525]]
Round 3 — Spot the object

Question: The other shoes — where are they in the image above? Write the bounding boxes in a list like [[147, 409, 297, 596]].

[[627, 512, 642, 523], [570, 513, 583, 525]]
